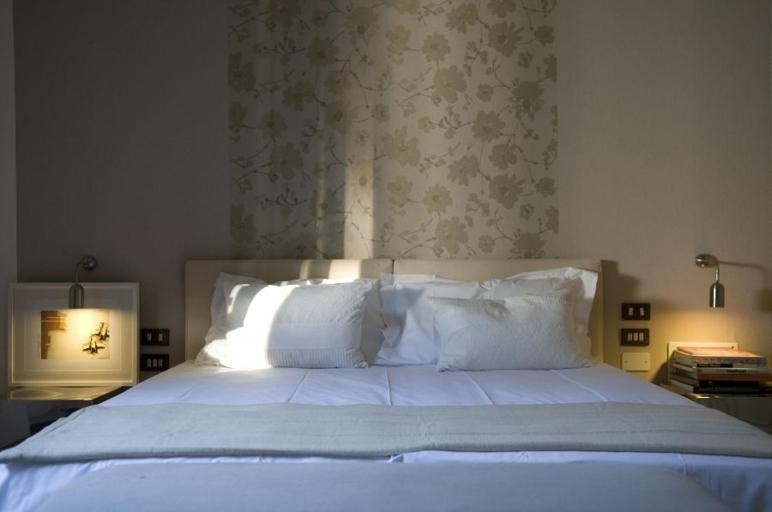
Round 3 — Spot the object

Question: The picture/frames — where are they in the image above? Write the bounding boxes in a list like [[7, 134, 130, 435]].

[[7, 281, 141, 387]]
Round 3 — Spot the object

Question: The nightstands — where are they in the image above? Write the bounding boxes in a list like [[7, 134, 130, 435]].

[[2, 385, 124, 451], [661, 383, 772, 435]]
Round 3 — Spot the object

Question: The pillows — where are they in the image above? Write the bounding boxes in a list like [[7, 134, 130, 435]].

[[425, 286, 597, 373], [220, 277, 373, 369], [374, 267, 600, 365], [215, 271, 386, 362]]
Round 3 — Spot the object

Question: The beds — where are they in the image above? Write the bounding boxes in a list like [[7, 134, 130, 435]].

[[0, 257, 772, 512]]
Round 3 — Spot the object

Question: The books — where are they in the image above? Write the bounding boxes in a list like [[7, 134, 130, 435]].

[[669, 345, 772, 397]]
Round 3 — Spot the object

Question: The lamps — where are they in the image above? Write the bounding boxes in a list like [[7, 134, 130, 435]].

[[696, 253, 725, 309], [68, 255, 97, 309]]
